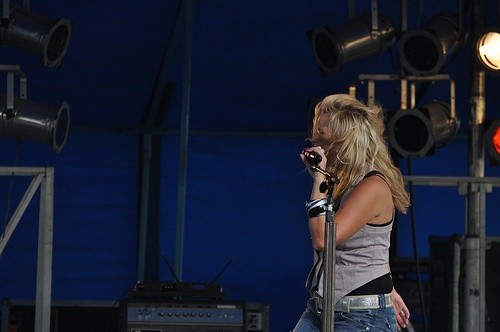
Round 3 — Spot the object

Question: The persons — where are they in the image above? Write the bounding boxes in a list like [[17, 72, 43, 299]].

[[293, 94, 410, 332]]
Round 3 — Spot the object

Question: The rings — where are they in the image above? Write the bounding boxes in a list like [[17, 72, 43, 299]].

[[400, 311, 405, 315]]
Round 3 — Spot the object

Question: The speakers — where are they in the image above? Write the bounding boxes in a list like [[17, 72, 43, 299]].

[[117, 299, 270, 332]]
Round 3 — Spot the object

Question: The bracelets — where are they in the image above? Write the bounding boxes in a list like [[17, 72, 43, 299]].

[[306, 198, 328, 218]]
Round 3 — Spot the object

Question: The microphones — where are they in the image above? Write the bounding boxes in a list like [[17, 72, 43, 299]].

[[302, 146, 322, 163]]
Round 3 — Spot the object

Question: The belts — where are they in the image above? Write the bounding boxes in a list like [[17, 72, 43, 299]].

[[312, 294, 393, 312]]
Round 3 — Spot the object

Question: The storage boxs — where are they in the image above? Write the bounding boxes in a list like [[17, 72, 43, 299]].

[[0, 298, 120, 332]]
